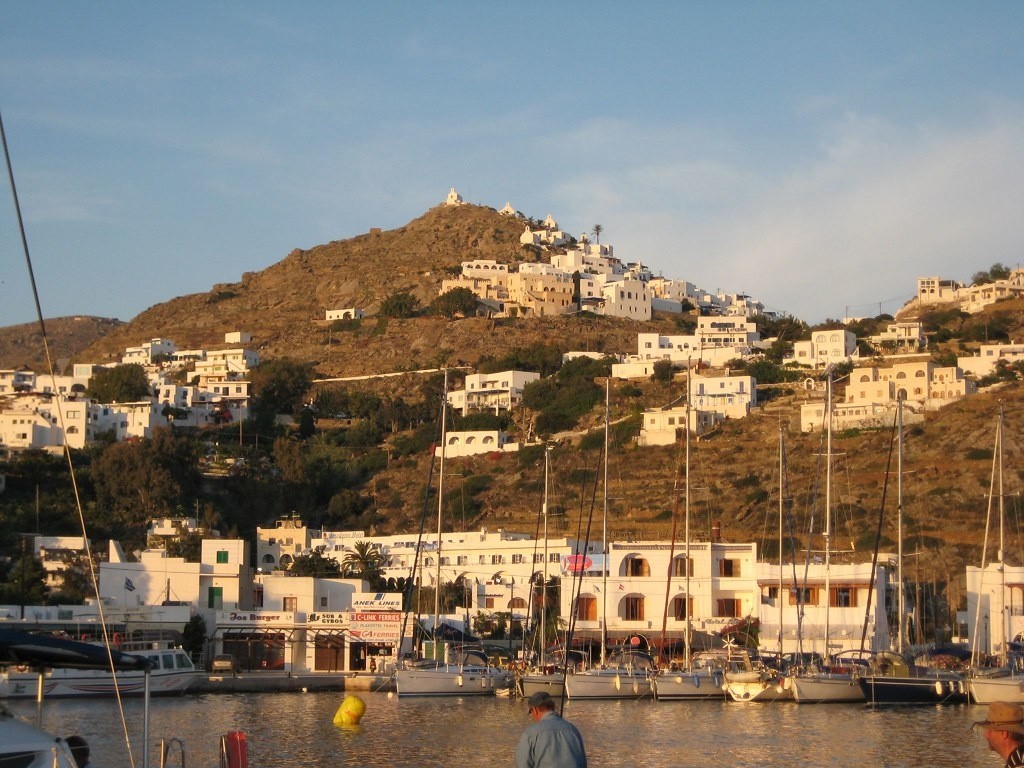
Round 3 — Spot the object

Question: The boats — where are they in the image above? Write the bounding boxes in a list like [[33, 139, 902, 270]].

[[0, 631, 207, 702]]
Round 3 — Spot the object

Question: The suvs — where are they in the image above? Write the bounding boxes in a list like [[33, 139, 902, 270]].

[[212, 654, 242, 673]]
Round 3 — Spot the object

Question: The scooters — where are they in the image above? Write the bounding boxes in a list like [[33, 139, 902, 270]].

[[370, 657, 377, 673]]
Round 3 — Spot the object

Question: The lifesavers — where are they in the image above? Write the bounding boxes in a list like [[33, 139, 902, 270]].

[[113, 632, 124, 646], [17, 664, 26, 672]]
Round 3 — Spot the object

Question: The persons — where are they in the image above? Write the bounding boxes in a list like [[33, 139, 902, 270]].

[[65, 736, 100, 768], [971, 701, 1024, 767], [125, 577, 135, 592], [515, 692, 587, 768]]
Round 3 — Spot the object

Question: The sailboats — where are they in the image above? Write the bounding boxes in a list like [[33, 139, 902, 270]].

[[515, 352, 1024, 703], [1, 116, 141, 768], [392, 368, 512, 694]]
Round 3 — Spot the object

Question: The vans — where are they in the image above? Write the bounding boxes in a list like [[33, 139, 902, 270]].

[[487, 655, 511, 671]]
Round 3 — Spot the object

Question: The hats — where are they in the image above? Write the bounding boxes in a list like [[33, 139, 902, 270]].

[[965, 701, 1024, 735], [527, 691, 555, 715]]
